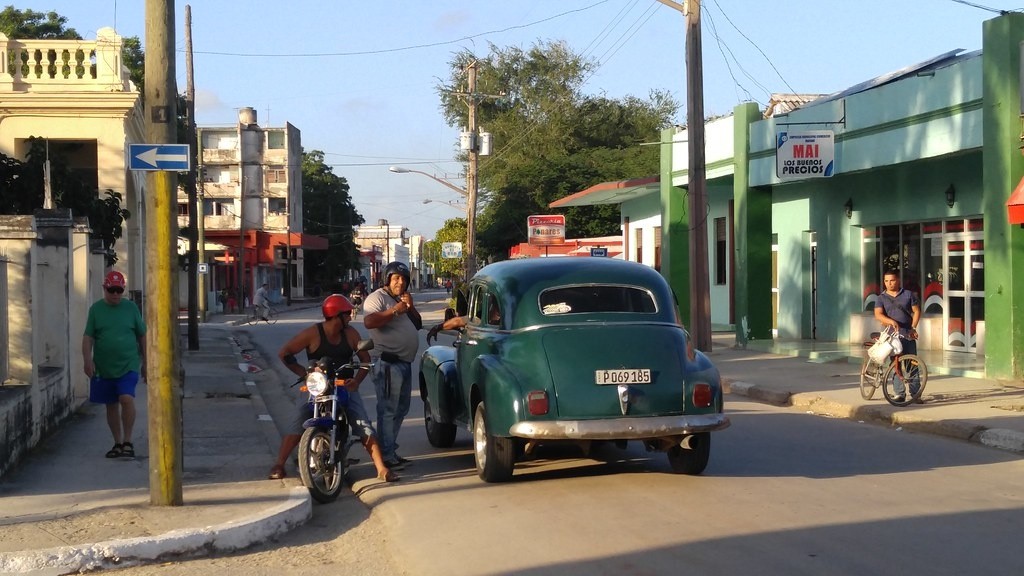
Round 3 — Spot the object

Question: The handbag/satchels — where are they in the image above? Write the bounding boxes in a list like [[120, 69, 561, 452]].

[[867, 324, 903, 365]]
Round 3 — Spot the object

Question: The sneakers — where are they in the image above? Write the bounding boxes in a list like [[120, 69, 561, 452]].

[[385, 451, 413, 471]]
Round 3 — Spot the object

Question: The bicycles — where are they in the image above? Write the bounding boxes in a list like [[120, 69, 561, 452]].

[[352, 304, 359, 321], [247, 305, 279, 326], [859, 331, 928, 408]]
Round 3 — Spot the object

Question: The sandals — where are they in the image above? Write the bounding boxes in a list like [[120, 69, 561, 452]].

[[105, 442, 134, 458]]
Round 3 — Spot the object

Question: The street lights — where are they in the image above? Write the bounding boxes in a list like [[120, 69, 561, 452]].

[[390, 165, 478, 284]]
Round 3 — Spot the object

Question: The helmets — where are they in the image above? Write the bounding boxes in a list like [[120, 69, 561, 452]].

[[382, 262, 411, 287], [321, 294, 355, 318]]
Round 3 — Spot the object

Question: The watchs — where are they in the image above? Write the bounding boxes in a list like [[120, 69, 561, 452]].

[[393, 308, 399, 317]]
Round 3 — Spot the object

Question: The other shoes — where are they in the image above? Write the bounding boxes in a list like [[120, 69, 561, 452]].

[[262, 317, 268, 321], [892, 397, 905, 401], [270, 465, 287, 478], [377, 473, 400, 481], [913, 399, 923, 404]]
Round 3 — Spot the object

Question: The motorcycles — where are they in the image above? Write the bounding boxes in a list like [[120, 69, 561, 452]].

[[289, 354, 376, 505]]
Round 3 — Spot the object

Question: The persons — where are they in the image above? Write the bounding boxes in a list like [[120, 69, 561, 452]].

[[81, 271, 148, 459], [874, 271, 925, 405], [268, 293, 401, 483], [252, 284, 271, 321], [446, 279, 452, 293], [426, 304, 502, 346], [363, 261, 423, 472], [350, 285, 363, 317]]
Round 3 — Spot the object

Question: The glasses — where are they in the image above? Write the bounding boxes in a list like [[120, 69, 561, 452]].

[[105, 286, 124, 294]]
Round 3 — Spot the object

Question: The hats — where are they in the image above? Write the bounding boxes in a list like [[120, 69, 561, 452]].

[[104, 271, 126, 288]]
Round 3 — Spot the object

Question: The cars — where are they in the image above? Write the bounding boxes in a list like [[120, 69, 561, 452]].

[[418, 255, 733, 483]]
